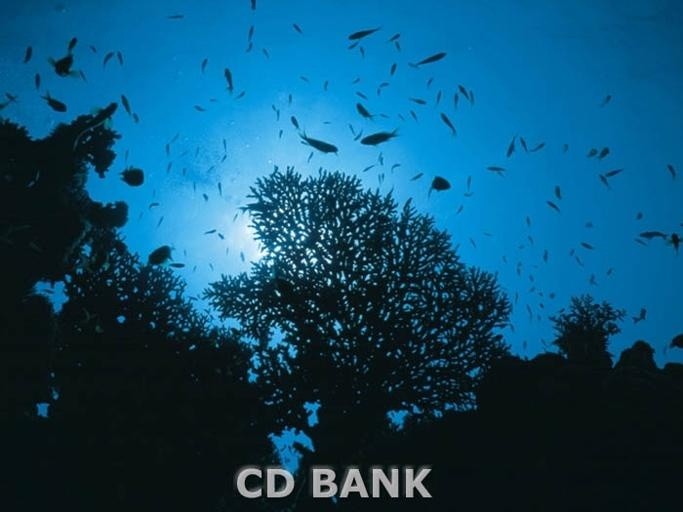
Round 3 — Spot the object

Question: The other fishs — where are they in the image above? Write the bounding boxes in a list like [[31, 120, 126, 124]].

[[1, 0, 683, 465]]
